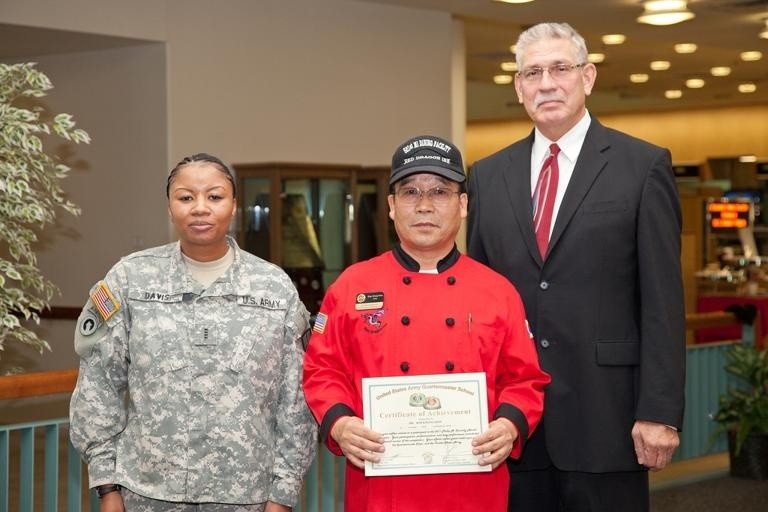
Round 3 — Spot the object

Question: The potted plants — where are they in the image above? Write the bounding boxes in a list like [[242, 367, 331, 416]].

[[705, 339, 767, 479]]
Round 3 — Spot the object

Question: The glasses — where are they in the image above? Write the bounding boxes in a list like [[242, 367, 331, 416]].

[[396, 186, 461, 201], [519, 63, 586, 81]]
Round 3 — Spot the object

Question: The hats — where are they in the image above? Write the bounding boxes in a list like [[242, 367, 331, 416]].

[[388, 135, 466, 185]]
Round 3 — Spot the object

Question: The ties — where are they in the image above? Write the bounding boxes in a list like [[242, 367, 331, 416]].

[[531, 143, 561, 262]]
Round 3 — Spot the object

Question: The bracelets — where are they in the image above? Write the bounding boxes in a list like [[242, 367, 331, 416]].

[[95, 484, 121, 497]]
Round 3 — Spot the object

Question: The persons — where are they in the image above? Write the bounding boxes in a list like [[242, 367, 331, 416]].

[[303, 135, 551, 512], [69, 154, 319, 512], [465, 23, 685, 512]]
